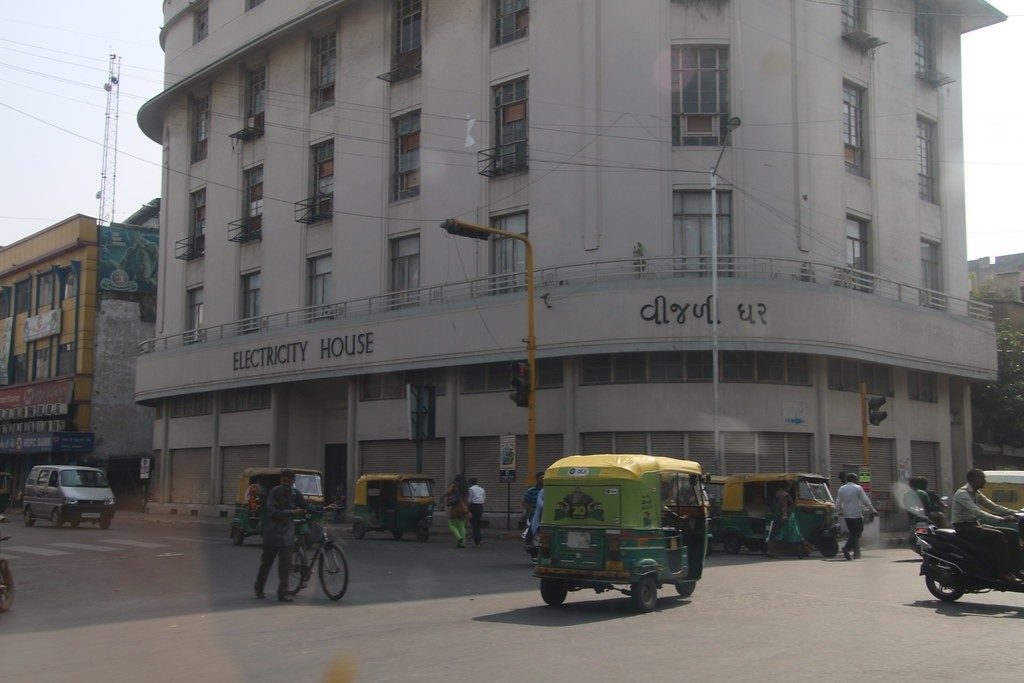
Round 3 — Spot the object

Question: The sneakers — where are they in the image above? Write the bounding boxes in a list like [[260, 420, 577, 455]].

[[1000, 572, 1022, 583]]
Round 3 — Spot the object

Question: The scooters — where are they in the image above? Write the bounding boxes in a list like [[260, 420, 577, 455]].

[[910, 495, 949, 554], [910, 508, 1024, 601]]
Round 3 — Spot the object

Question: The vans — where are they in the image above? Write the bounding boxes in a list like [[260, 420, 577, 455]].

[[23, 464, 116, 529]]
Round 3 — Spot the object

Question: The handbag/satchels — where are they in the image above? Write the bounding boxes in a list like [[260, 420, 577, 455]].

[[446, 483, 461, 505]]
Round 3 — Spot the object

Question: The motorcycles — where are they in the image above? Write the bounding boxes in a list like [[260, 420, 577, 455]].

[[534, 454, 710, 613], [709, 475, 721, 542], [231, 468, 326, 549], [0, 472, 13, 513], [352, 474, 436, 541], [721, 474, 841, 558], [961, 471, 1024, 510]]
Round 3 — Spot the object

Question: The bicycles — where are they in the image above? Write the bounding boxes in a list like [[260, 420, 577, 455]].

[[286, 506, 349, 599]]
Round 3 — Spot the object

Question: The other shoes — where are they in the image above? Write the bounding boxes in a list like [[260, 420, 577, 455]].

[[457, 539, 467, 549], [278, 590, 292, 601], [798, 550, 809, 558], [254, 583, 265, 599], [841, 548, 863, 560], [475, 541, 484, 548], [766, 551, 779, 559], [532, 556, 538, 565]]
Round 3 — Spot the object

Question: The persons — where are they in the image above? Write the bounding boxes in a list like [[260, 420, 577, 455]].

[[835, 473, 878, 560], [951, 469, 1024, 583], [440, 474, 468, 548], [521, 472, 544, 563], [254, 469, 337, 602], [467, 477, 486, 548], [660, 480, 688, 530], [903, 477, 949, 550], [766, 480, 811, 559]]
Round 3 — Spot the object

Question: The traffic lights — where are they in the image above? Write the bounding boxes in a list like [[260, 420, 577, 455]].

[[869, 396, 887, 425], [510, 362, 528, 406]]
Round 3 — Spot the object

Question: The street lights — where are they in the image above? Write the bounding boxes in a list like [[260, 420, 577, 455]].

[[708, 117, 743, 471]]
